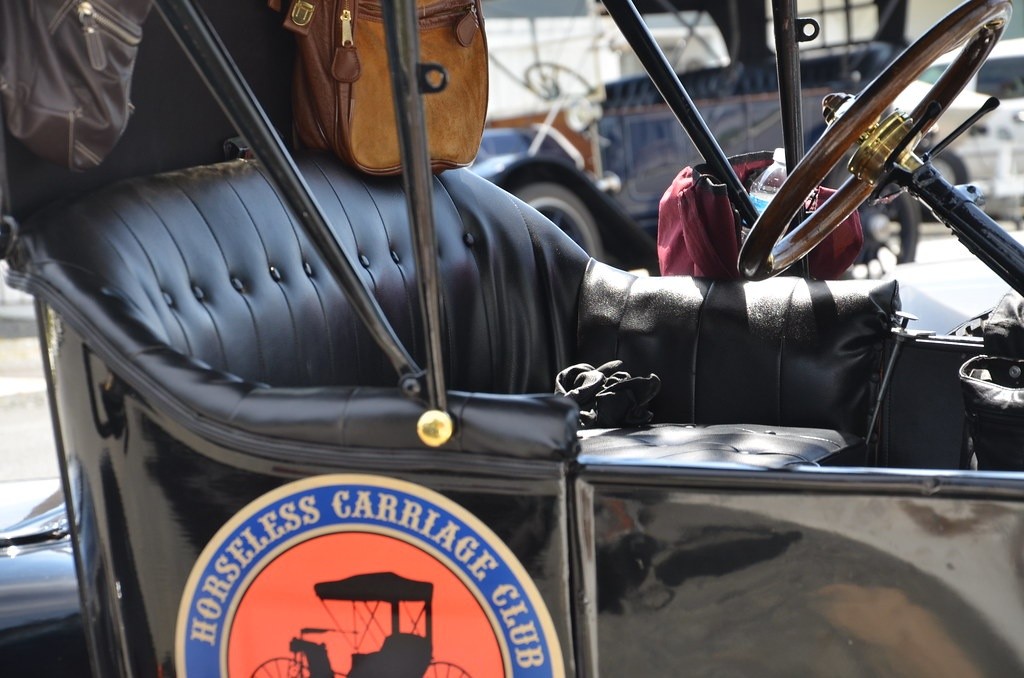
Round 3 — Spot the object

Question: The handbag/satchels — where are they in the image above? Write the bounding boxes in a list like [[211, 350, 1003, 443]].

[[656, 151, 862, 281], [1, 1, 154, 175], [283, 0, 489, 176]]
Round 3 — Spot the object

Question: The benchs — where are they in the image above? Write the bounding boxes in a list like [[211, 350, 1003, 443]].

[[49, 144, 902, 471]]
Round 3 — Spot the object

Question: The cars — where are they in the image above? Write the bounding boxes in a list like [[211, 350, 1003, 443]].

[[906, 36, 1024, 232]]
